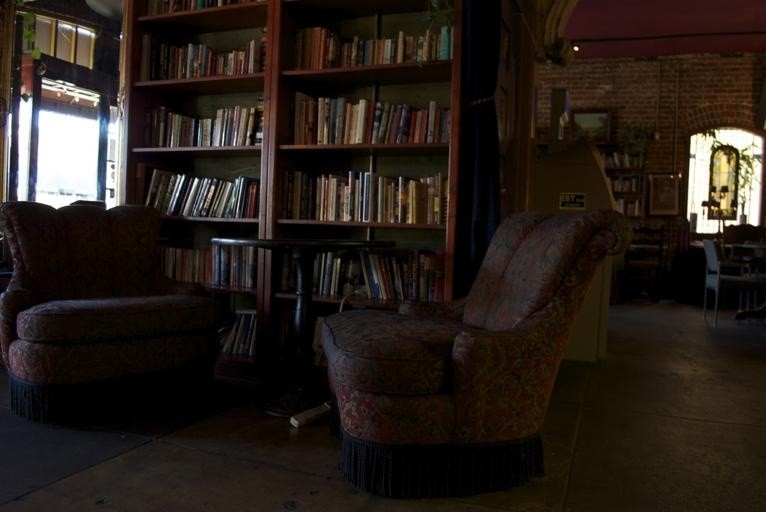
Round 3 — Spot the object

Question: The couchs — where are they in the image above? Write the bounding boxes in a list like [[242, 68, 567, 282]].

[[0, 199, 226, 432], [316, 206, 633, 500]]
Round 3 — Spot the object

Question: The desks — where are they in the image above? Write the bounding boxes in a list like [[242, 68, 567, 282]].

[[211, 238, 396, 418], [690, 242, 766, 320]]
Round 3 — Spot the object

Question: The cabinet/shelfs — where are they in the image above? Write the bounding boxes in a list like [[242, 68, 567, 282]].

[[122, 0, 273, 332], [566, 249, 612, 364], [606, 158, 649, 229], [262, 0, 509, 329]]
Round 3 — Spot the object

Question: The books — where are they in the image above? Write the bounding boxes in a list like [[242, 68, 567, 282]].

[[155, 238, 258, 302], [281, 168, 448, 225], [223, 309, 257, 356], [289, 90, 452, 145], [280, 242, 446, 302], [138, 1, 268, 82], [293, 1, 454, 71], [143, 95, 264, 219], [312, 317, 329, 369], [603, 152, 644, 216]]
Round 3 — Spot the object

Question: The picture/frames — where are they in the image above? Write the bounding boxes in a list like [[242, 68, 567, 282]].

[[569, 107, 612, 146]]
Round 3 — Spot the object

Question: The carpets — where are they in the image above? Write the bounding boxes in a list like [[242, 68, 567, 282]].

[[0, 379, 252, 506]]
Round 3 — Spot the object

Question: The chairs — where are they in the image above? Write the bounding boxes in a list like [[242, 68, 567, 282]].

[[703, 238, 757, 329]]
